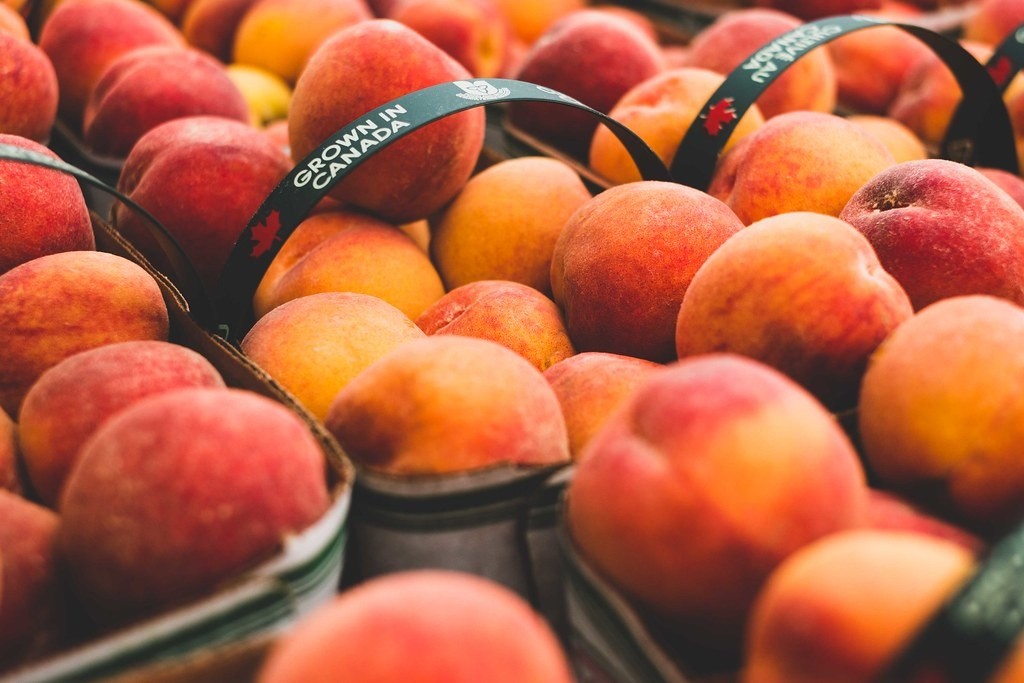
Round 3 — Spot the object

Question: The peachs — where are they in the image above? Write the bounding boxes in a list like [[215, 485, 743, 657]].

[[0, 0, 1024, 683]]
